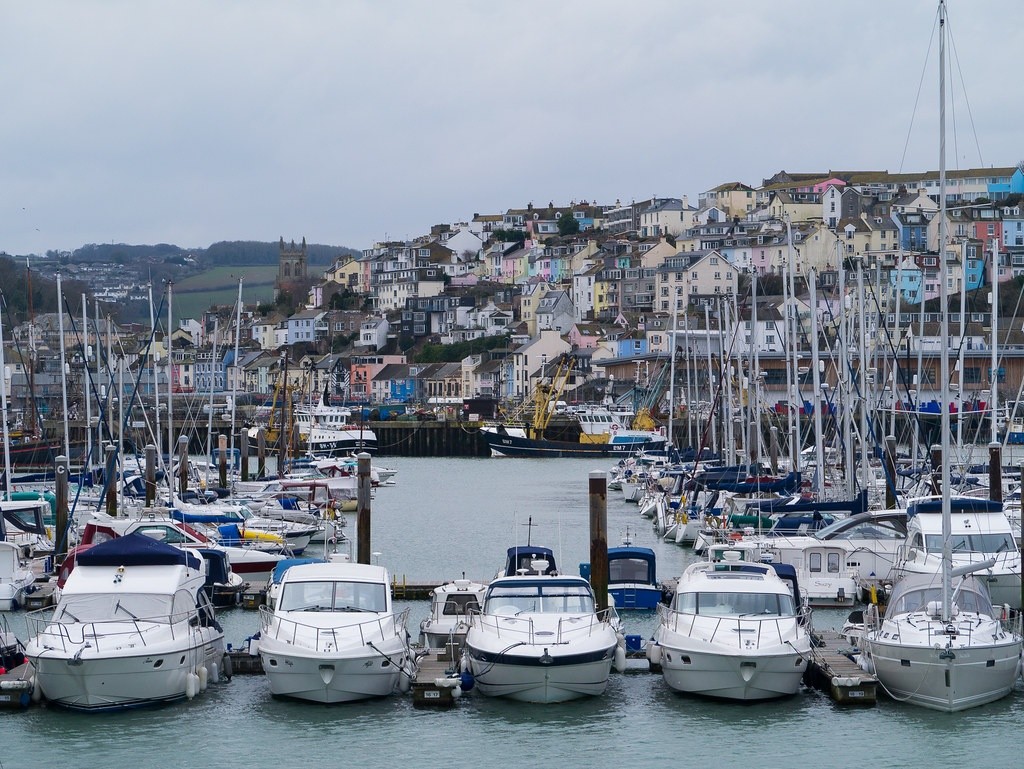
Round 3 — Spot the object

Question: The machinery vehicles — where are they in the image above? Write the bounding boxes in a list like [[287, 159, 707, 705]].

[[248, 364, 310, 447]]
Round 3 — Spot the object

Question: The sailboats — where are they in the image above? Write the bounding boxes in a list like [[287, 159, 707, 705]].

[[859, 1, 1024, 713], [158, 278, 325, 555], [217, 278, 286, 551], [57, 357, 287, 589]]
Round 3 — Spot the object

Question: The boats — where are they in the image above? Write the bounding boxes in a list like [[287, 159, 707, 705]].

[[692, 511, 851, 554], [674, 497, 773, 544], [504, 515, 559, 575], [479, 350, 668, 457], [708, 510, 909, 601], [645, 551, 813, 703], [257, 562, 409, 704], [897, 496, 1024, 609], [293, 380, 378, 457], [20, 531, 226, 712], [465, 574, 618, 704], [418, 572, 489, 648], [309, 457, 398, 482], [621, 471, 687, 502], [234, 474, 377, 511], [578, 525, 664, 610]]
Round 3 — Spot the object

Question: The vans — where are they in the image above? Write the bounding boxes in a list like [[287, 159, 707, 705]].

[[548, 401, 567, 415]]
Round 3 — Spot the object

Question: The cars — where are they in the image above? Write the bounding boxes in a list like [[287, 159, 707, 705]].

[[567, 406, 578, 414]]
